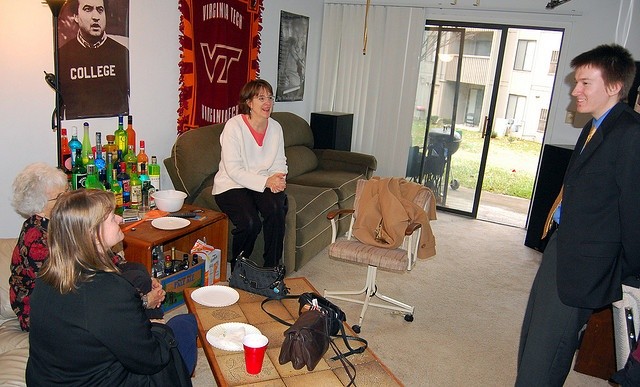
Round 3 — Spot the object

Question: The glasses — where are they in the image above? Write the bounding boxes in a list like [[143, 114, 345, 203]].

[[252, 95, 276, 102], [47, 185, 72, 202]]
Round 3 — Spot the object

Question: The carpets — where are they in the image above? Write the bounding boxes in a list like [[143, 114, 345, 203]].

[[162, 210, 620, 386]]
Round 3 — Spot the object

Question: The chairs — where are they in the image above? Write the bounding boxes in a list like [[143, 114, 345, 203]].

[[323, 179, 433, 334]]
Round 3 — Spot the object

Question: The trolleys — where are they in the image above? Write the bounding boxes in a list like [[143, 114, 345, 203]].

[[429, 124, 461, 190]]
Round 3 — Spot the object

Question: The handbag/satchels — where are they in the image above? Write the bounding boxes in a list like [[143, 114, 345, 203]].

[[260, 291, 368, 361], [226, 255, 290, 300], [278, 309, 357, 386]]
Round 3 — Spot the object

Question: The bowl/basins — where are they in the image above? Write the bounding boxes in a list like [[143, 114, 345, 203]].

[[151, 189, 187, 212]]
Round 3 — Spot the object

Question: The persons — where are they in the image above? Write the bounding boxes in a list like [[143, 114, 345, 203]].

[[26, 190, 199, 387], [8, 163, 69, 332], [513, 43, 636, 387], [211, 79, 290, 275]]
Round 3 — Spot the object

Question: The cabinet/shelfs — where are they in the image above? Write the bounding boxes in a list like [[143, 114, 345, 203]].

[[98, 205, 228, 316], [524, 143, 576, 252], [311, 111, 353, 152]]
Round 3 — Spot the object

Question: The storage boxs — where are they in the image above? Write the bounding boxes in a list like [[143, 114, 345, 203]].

[[152, 249, 205, 317]]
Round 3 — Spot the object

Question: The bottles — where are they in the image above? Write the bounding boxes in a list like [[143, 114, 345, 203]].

[[95, 144, 105, 171], [125, 114, 137, 150], [98, 169, 111, 193], [183, 253, 190, 267], [192, 254, 198, 266], [143, 180, 155, 209], [123, 144, 138, 178], [114, 150, 125, 173], [68, 126, 82, 167], [87, 164, 105, 191], [140, 163, 149, 186], [103, 135, 117, 154], [82, 122, 94, 165], [136, 140, 148, 163], [72, 149, 86, 189], [171, 247, 176, 260], [160, 245, 166, 257], [60, 128, 72, 180], [151, 252, 164, 278], [117, 161, 129, 210], [87, 154, 94, 166], [127, 163, 143, 208], [109, 169, 124, 216], [155, 245, 160, 253], [114, 114, 130, 154], [106, 152, 114, 187], [163, 254, 172, 272], [96, 131, 102, 152], [149, 155, 160, 192]]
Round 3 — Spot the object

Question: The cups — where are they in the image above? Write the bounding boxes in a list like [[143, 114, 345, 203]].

[[242, 334, 269, 375]]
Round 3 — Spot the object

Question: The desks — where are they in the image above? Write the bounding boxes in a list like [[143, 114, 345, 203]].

[[183, 277, 405, 386]]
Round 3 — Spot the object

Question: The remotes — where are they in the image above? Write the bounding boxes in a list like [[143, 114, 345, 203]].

[[168, 212, 197, 217]]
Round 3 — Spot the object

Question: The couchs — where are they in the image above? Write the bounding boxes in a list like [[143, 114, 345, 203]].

[[0, 238, 43, 387], [163, 111, 377, 277]]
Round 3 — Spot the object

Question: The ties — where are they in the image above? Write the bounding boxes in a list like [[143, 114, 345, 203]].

[[540, 126, 598, 241]]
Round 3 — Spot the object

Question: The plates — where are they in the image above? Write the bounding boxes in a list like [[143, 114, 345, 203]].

[[190, 284, 240, 308], [206, 321, 262, 351], [151, 217, 191, 230]]
